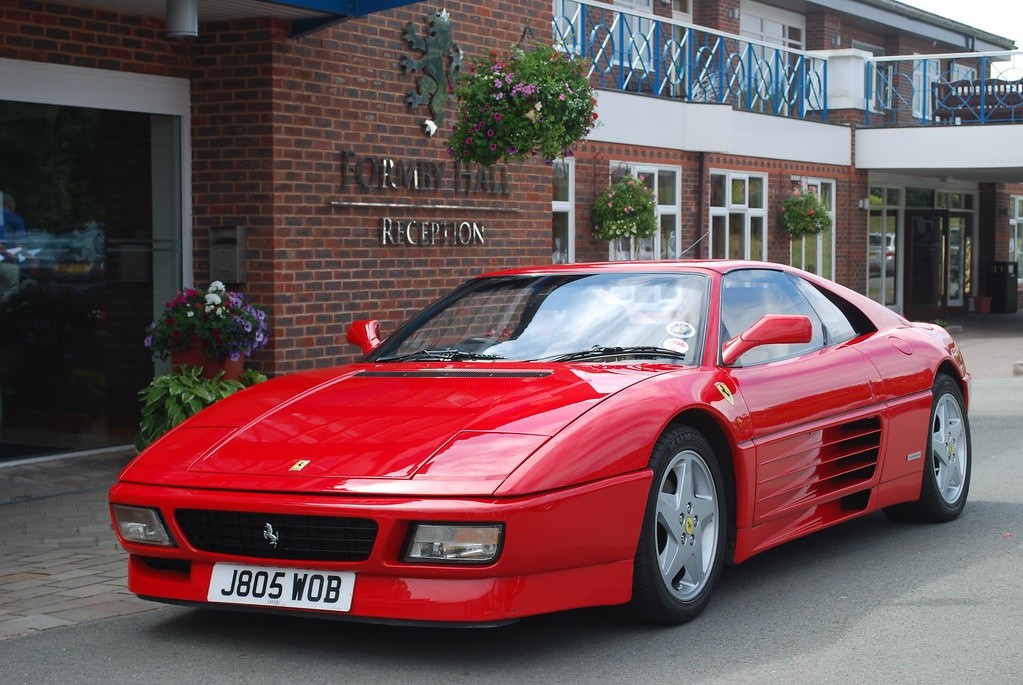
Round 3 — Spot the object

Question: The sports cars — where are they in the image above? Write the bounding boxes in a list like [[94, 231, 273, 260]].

[[107, 261, 971, 626]]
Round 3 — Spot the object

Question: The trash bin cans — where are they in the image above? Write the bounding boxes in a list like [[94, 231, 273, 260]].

[[987, 261, 1018, 313]]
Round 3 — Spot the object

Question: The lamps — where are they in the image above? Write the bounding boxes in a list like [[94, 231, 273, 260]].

[[831, 33, 841, 48], [661, 0, 671, 5], [729, 7, 738, 19]]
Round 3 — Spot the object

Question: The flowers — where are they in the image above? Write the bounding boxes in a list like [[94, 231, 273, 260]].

[[591, 176, 661, 241], [143, 278, 273, 364], [444, 40, 601, 171], [776, 184, 833, 241]]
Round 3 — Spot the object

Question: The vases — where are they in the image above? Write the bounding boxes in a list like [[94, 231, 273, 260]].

[[166, 330, 245, 386]]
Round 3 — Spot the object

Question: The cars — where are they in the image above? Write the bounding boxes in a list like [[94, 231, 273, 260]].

[[869, 232, 896, 272], [18, 224, 152, 288]]
